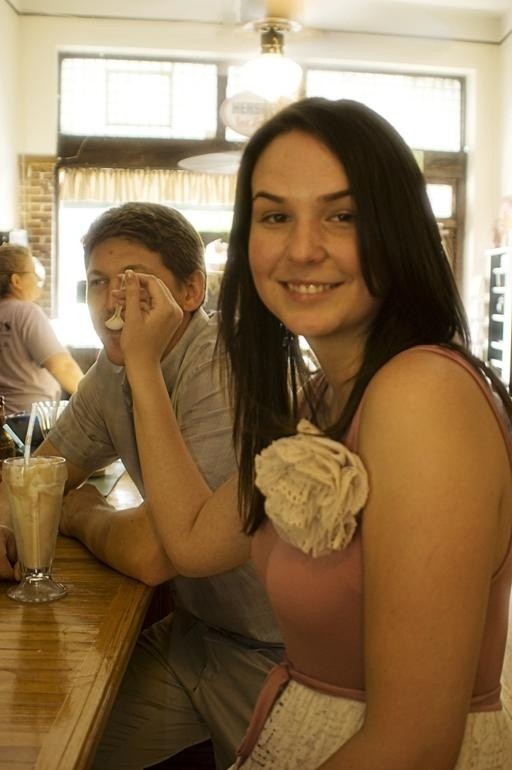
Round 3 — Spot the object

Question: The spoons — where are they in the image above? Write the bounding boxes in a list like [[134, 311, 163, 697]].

[[102, 273, 134, 331]]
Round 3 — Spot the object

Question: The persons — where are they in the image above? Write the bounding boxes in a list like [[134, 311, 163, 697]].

[[110, 95, 511, 769], [0, 200, 289, 765], [0, 242, 85, 458]]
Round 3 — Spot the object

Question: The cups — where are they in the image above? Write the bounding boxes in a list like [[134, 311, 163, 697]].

[[3, 454, 73, 603]]
